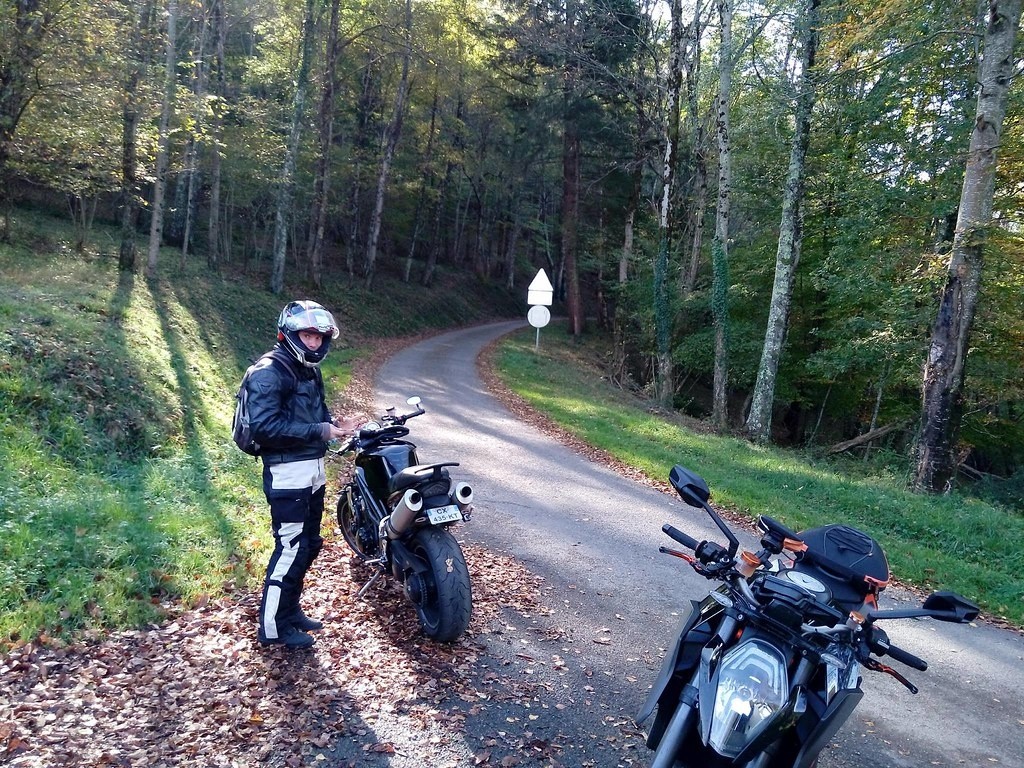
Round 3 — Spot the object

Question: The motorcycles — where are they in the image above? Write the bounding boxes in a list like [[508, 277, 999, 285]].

[[632, 464, 981, 768], [326, 395, 477, 644]]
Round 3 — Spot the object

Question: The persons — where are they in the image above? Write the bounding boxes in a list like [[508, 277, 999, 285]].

[[246, 300, 368, 650]]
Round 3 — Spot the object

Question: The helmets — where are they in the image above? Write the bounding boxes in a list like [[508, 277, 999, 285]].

[[277, 301, 334, 368]]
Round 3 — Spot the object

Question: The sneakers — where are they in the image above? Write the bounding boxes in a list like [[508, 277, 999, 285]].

[[256, 628, 316, 649], [290, 609, 322, 630]]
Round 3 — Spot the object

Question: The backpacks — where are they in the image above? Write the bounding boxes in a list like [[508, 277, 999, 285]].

[[231, 350, 321, 457]]
